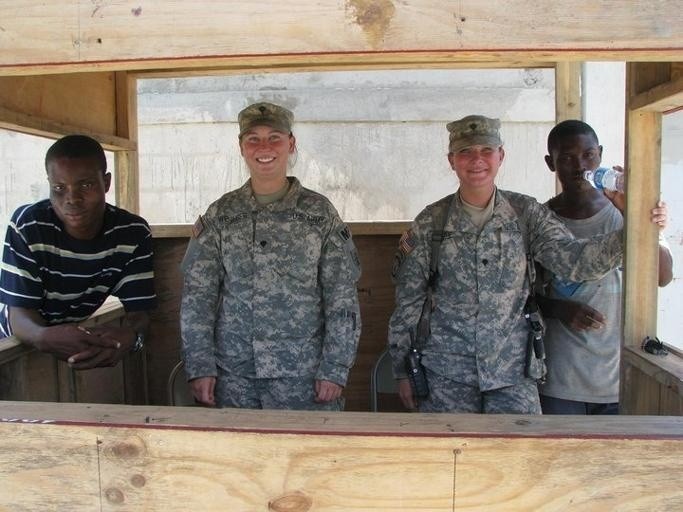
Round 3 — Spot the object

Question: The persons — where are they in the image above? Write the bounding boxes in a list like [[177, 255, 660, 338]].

[[0, 133, 157, 371], [178, 101, 363, 412], [385, 114, 669, 416], [526, 119, 674, 415]]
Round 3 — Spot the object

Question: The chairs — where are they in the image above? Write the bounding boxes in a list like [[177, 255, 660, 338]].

[[370, 344, 417, 415]]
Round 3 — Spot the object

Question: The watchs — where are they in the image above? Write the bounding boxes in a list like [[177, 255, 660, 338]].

[[130, 328, 145, 353]]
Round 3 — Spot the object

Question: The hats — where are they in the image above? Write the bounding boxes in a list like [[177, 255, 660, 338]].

[[238, 102, 294, 139], [446, 115, 503, 153]]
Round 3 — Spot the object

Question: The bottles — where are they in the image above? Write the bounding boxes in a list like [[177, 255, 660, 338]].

[[582, 165, 624, 195]]
